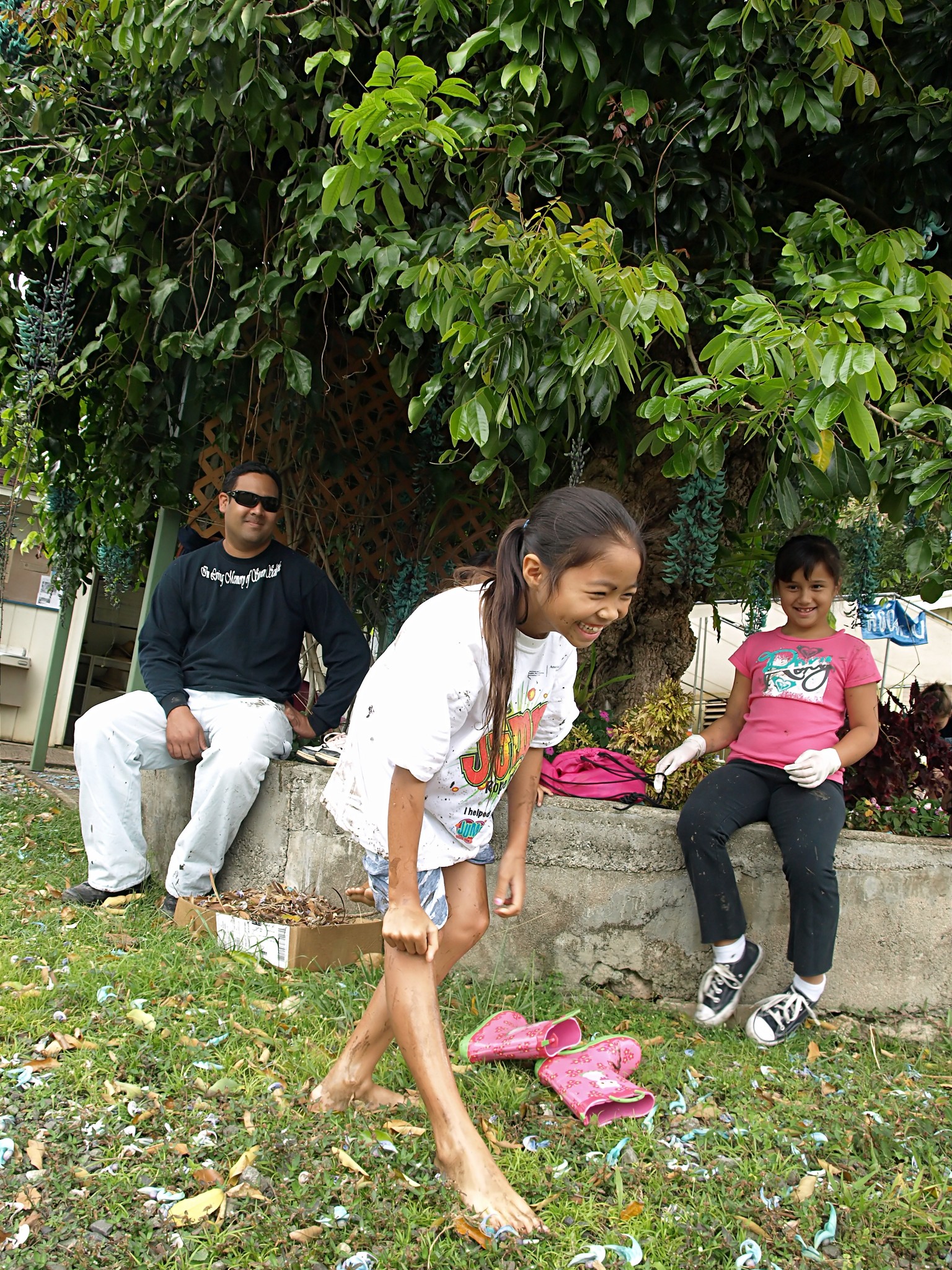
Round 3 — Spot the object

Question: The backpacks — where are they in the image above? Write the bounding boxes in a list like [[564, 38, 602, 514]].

[[539, 747, 679, 810]]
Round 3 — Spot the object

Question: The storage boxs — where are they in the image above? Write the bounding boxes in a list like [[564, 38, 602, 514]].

[[174, 895, 383, 974]]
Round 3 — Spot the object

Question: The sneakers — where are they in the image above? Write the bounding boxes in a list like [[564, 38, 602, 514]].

[[694, 940, 764, 1026], [314, 740, 345, 766], [61, 881, 143, 904], [745, 982, 820, 1046], [296, 732, 347, 763], [161, 892, 179, 918]]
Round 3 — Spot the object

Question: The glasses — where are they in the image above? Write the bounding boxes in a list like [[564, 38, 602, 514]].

[[226, 490, 282, 513]]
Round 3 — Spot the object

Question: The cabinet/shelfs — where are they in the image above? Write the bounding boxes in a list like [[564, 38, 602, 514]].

[[69, 653, 131, 717]]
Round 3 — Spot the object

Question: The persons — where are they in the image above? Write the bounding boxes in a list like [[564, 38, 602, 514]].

[[61, 462, 374, 921], [310, 484, 646, 1235], [656, 533, 882, 1047]]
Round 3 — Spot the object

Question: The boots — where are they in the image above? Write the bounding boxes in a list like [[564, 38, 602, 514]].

[[534, 1034, 655, 1128], [460, 1010, 589, 1062]]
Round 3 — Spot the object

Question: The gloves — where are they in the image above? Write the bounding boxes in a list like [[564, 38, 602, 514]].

[[654, 735, 706, 793], [784, 748, 841, 788]]
